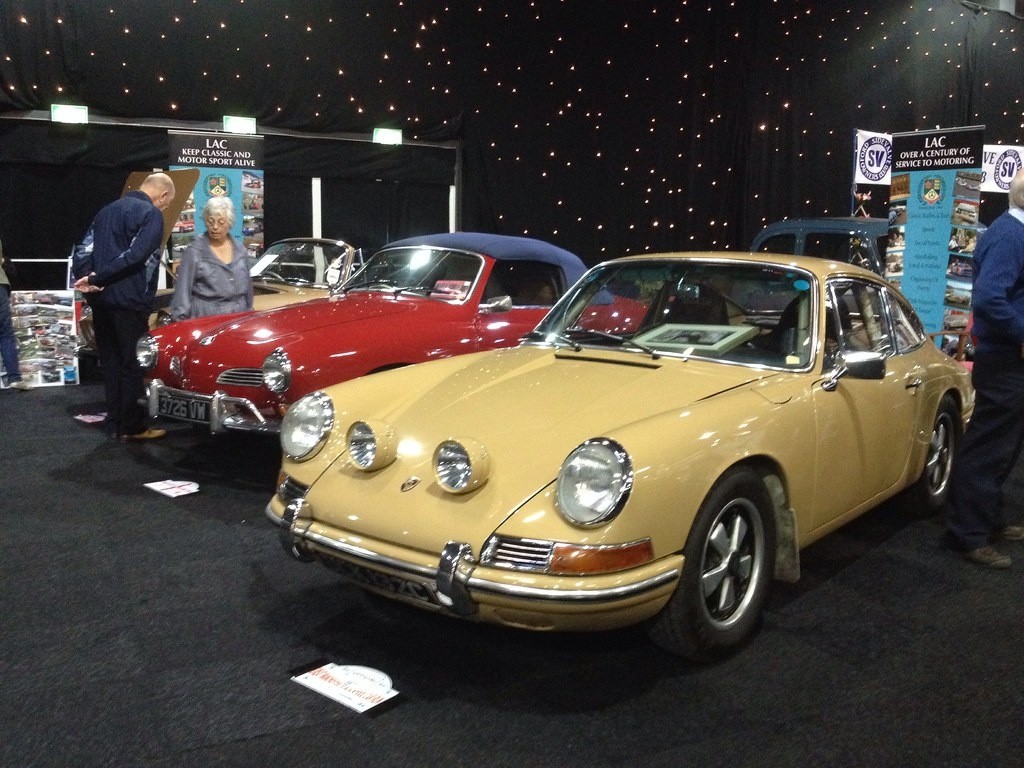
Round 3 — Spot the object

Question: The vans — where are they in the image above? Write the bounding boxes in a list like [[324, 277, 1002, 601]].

[[755, 217, 890, 278]]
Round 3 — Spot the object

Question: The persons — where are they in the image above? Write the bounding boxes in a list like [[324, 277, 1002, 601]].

[[73, 173, 177, 440], [170, 196, 253, 322], [949, 167, 1024, 569], [0, 239, 23, 387]]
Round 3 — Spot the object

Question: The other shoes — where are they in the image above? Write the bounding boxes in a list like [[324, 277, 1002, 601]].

[[965, 548, 1008, 569], [121, 428, 166, 442], [1001, 525, 1024, 544]]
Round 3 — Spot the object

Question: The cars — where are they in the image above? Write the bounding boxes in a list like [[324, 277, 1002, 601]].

[[247, 244, 261, 259], [171, 219, 195, 233], [243, 223, 262, 236], [265, 249, 976, 667], [79, 236, 356, 336], [244, 179, 261, 188], [244, 200, 258, 209], [135, 232, 650, 431]]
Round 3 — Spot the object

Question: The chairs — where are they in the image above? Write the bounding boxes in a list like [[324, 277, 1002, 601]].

[[767, 291, 851, 362], [658, 287, 729, 330], [412, 259, 448, 285]]
